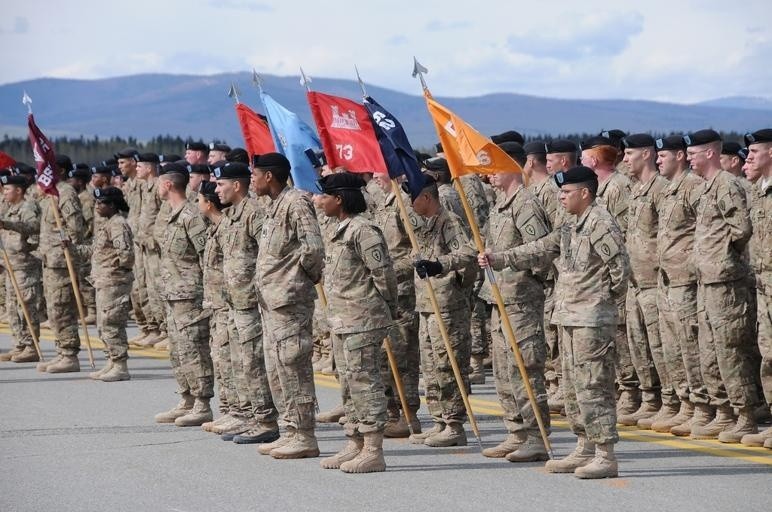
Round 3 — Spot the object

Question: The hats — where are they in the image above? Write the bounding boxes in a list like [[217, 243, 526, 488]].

[[491, 130, 747, 161], [253, 152, 291, 168], [426, 158, 448, 170], [744, 128, 772, 147], [554, 166, 598, 188], [317, 173, 361, 192], [0, 141, 251, 200], [400, 175, 435, 194]]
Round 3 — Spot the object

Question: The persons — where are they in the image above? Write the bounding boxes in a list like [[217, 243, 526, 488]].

[[114, 142, 398, 472], [372, 132, 631, 478], [0, 157, 135, 381], [616, 131, 771, 446]]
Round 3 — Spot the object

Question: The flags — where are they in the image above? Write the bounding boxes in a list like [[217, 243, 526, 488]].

[[234, 104, 275, 168], [425, 88, 526, 181], [307, 89, 387, 175], [364, 94, 425, 202], [27, 113, 61, 199], [260, 92, 325, 194]]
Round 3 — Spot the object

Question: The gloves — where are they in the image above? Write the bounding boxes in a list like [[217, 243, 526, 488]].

[[415, 260, 442, 280]]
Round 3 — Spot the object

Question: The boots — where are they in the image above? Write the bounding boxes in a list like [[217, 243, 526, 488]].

[[545, 437, 596, 472], [320, 434, 386, 473], [156, 397, 213, 426], [468, 357, 485, 384], [614, 391, 772, 449], [89, 359, 130, 381], [574, 445, 617, 479], [384, 410, 421, 438]]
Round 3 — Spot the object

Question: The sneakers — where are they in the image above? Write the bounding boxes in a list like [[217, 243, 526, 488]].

[[37, 355, 80, 373], [311, 358, 337, 375], [127, 333, 169, 351], [408, 425, 467, 447], [0, 344, 41, 362], [316, 405, 347, 425], [78, 314, 96, 324], [483, 446, 513, 457], [201, 415, 280, 444], [256, 432, 320, 459], [505, 442, 552, 462]]
